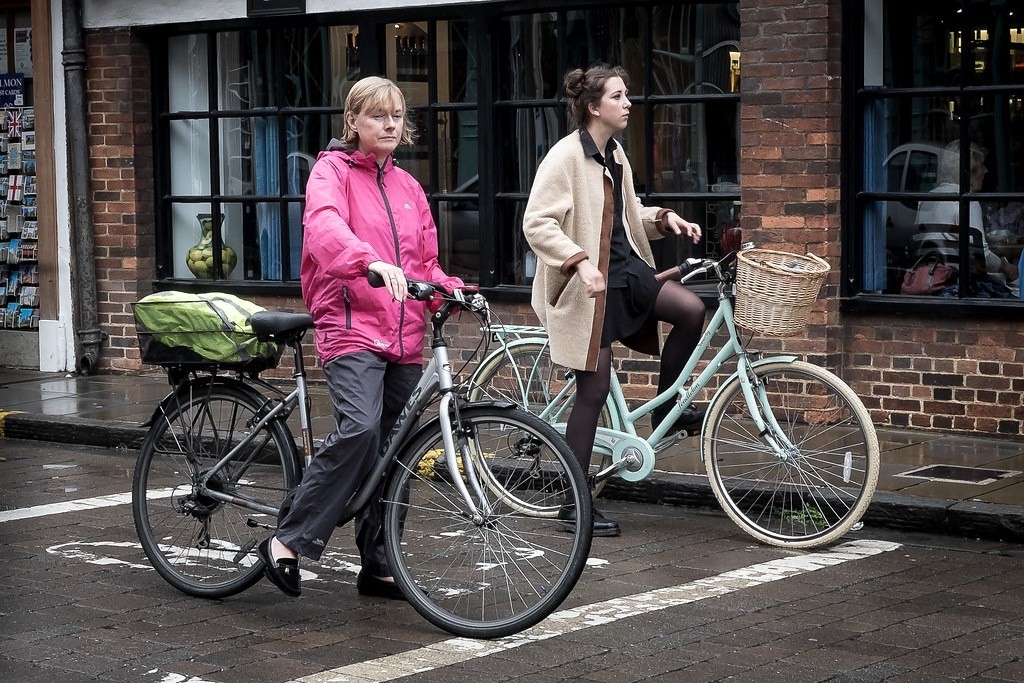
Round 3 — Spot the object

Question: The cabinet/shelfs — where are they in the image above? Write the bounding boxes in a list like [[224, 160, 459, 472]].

[[330, 21, 450, 274]]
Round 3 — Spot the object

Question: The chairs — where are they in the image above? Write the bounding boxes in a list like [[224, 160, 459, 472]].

[[896, 225, 987, 298]]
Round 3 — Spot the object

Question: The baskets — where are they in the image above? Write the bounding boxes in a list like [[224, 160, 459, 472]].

[[733, 249, 831, 338], [130, 300, 286, 368]]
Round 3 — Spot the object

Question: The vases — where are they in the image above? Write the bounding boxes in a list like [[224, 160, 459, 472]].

[[186, 214, 238, 279]]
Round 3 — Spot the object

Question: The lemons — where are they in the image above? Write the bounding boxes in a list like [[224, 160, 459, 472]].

[[188, 221, 235, 278]]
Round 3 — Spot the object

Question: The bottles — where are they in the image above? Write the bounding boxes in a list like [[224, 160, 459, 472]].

[[345, 30, 430, 84], [186, 214, 238, 280]]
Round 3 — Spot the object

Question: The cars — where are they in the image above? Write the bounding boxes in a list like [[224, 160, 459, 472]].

[[229, 152, 318, 220], [431, 175, 481, 244], [884, 143, 948, 240]]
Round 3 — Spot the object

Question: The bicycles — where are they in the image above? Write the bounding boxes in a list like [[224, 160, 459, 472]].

[[467, 242, 879, 551], [130, 269, 593, 640]]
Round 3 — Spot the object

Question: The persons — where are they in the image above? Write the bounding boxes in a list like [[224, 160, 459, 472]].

[[913, 138, 1024, 299], [256, 76, 490, 600], [523, 67, 705, 537]]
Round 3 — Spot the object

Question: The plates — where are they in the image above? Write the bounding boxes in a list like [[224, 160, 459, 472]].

[[988, 229, 1011, 242]]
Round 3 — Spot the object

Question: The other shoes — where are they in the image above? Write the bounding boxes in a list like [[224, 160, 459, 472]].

[[357, 571, 430, 600], [256, 533, 301, 597]]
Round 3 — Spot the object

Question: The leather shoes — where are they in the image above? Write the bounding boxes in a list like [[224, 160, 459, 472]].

[[651, 405, 710, 433], [556, 503, 621, 537]]
